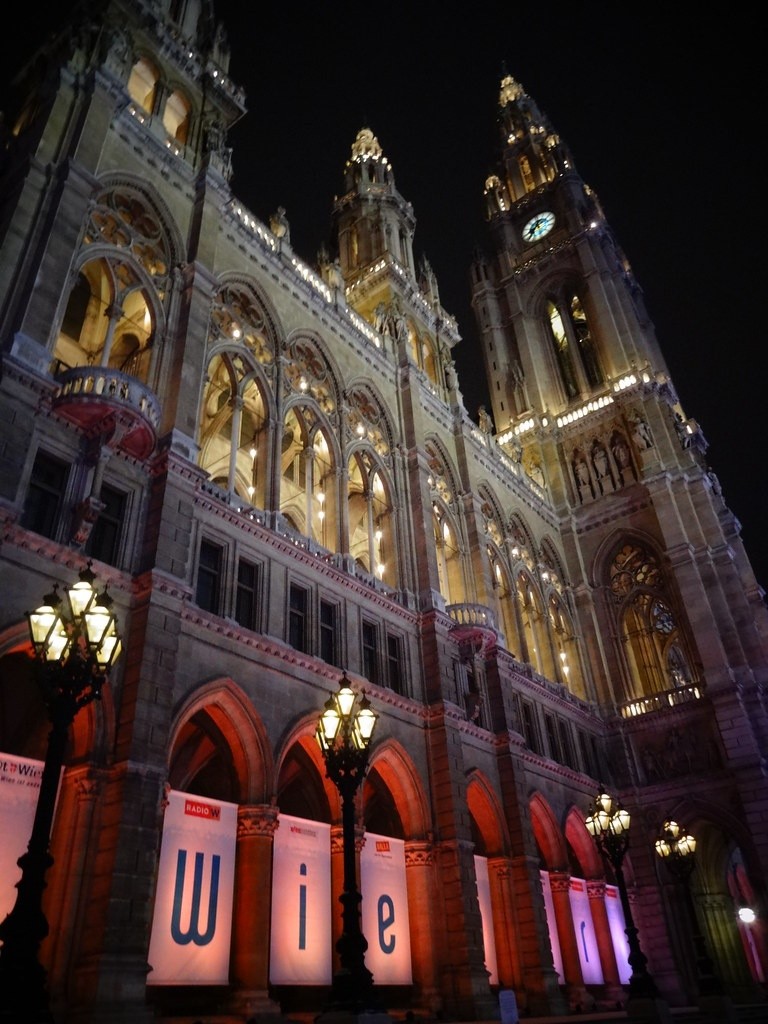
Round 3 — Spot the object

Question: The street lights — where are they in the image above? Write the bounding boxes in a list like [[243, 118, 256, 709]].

[[0, 560, 125, 1023], [654, 817, 739, 1024], [583, 781, 674, 1024], [311, 669, 398, 1024]]
